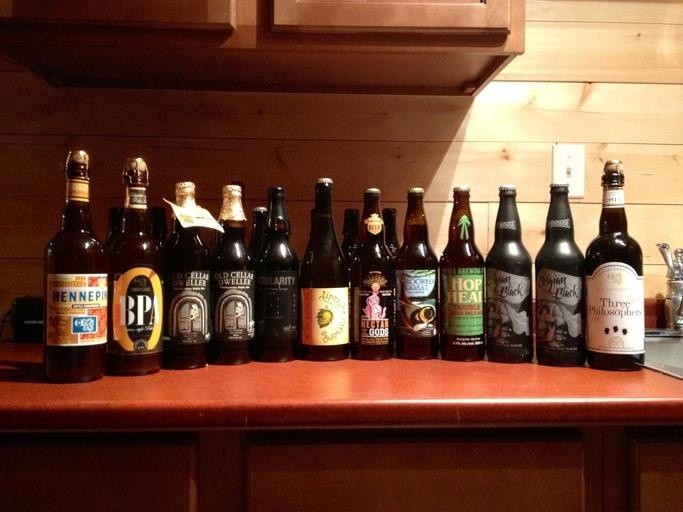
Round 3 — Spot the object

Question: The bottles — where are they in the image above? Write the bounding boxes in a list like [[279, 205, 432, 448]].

[[535, 183, 584, 367], [382, 208, 400, 254], [106, 207, 123, 247], [251, 184, 298, 363], [42, 151, 108, 383], [297, 177, 352, 362], [341, 207, 361, 256], [485, 185, 534, 364], [162, 182, 212, 370], [584, 159, 646, 372], [249, 207, 268, 258], [394, 187, 440, 360], [438, 186, 485, 361], [108, 156, 163, 376], [207, 185, 257, 366], [351, 188, 395, 361], [151, 204, 168, 241]]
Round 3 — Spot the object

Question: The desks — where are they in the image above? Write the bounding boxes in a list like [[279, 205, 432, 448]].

[[0, 333, 682, 512]]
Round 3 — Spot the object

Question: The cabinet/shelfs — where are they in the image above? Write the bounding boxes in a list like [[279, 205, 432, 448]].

[[0, 427, 682, 512], [0, 1, 525, 98]]
